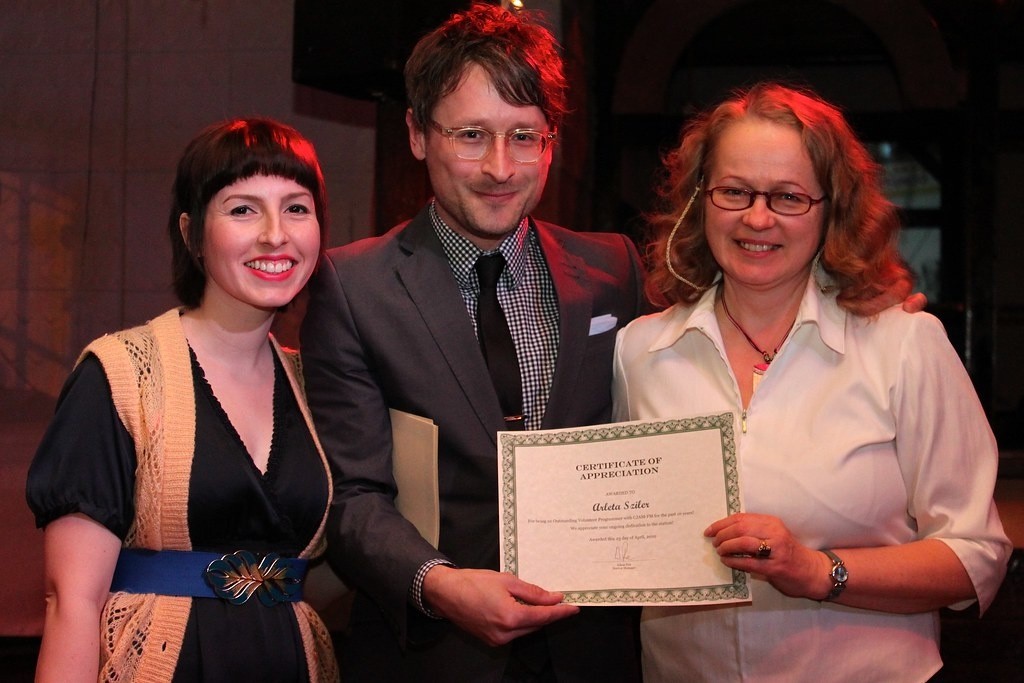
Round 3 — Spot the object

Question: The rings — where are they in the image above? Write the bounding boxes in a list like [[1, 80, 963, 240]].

[[753, 539, 771, 559]]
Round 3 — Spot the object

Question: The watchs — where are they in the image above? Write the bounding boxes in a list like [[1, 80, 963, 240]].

[[816, 548, 848, 604]]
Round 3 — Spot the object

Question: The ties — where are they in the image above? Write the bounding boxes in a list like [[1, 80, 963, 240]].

[[476, 253, 525, 432]]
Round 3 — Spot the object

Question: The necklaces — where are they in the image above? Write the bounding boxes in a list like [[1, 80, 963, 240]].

[[720, 279, 797, 395]]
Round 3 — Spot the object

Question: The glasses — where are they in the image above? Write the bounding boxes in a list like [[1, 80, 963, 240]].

[[704, 184, 827, 217], [422, 115, 557, 164]]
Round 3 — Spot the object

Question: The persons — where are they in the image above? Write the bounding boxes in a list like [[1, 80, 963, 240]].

[[298, 4, 928, 682], [610, 82, 1013, 683], [25, 119, 343, 682]]
